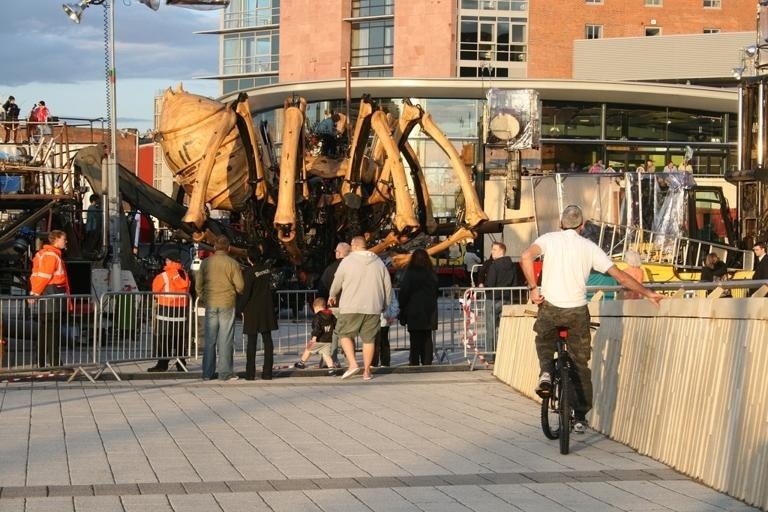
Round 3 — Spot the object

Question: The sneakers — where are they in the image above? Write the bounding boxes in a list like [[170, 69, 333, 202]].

[[245, 375, 255, 380], [574, 420, 586, 433], [261, 374, 272, 379], [328, 370, 336, 376], [225, 376, 240, 380], [534, 372, 552, 394], [295, 362, 305, 369], [148, 365, 168, 372], [203, 373, 218, 380], [177, 366, 184, 371]]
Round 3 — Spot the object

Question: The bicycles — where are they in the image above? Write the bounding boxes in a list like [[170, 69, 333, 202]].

[[522, 305, 604, 458]]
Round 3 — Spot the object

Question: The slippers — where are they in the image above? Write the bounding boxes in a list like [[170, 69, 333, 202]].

[[341, 368, 361, 379], [363, 374, 374, 380]]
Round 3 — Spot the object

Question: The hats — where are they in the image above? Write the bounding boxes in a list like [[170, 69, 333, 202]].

[[561, 205, 583, 229], [160, 249, 179, 259]]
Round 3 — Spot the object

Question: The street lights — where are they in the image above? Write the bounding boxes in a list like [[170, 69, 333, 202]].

[[727, 44, 757, 174], [62, 1, 164, 294]]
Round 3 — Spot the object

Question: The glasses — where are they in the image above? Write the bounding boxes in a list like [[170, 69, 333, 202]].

[[334, 249, 340, 252]]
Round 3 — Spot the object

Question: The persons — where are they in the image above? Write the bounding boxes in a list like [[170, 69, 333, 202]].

[[2, 96, 20, 144], [34, 102, 49, 143], [477, 240, 542, 367], [25, 193, 439, 381], [522, 158, 693, 176], [520, 204, 666, 432], [587, 243, 767, 300]]
